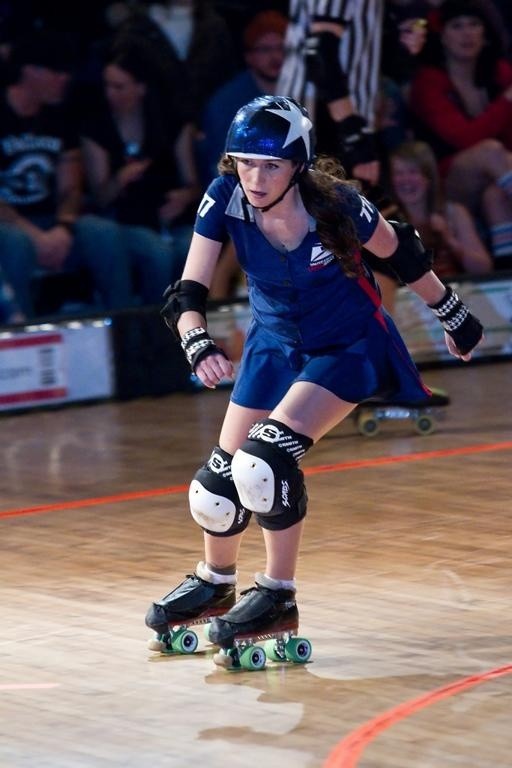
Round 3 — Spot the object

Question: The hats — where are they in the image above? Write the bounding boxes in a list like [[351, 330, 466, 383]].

[[243, 10, 288, 47]]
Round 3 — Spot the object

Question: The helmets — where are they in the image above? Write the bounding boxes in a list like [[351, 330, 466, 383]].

[[225, 95, 317, 169]]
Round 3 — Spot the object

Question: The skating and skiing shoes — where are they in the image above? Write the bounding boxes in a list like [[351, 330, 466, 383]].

[[209, 571, 311, 671], [145, 561, 238, 656], [354, 387, 449, 437]]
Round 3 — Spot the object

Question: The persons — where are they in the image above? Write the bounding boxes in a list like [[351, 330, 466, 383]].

[[146, 97, 484, 646], [1, 1, 511, 327]]
